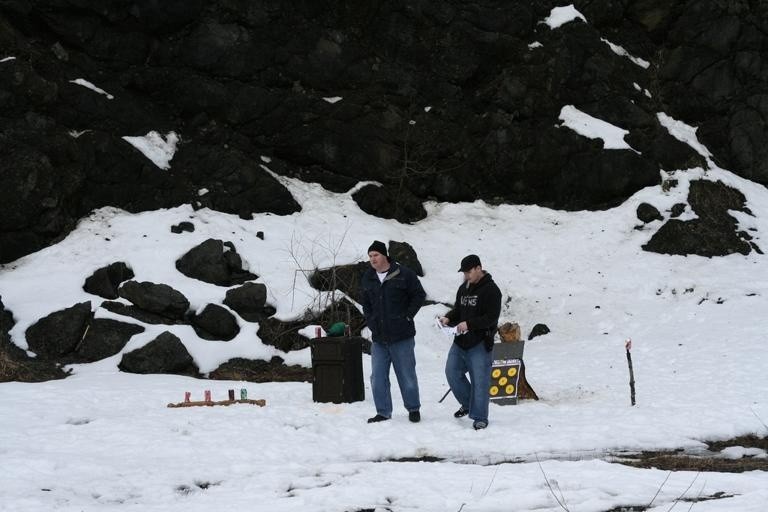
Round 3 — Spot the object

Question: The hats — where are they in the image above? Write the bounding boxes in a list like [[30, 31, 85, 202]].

[[368, 241, 387, 256], [458, 254, 480, 272]]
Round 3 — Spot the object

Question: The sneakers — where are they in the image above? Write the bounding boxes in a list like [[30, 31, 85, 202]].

[[473, 421, 487, 429], [454, 407, 470, 418], [367, 415, 387, 423], [409, 412, 420, 422]]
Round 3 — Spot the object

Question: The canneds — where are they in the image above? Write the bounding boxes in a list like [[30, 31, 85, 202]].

[[241, 389, 248, 400], [185, 391, 191, 403], [315, 327, 321, 338], [344, 324, 350, 337], [204, 390, 211, 401], [228, 389, 234, 401]]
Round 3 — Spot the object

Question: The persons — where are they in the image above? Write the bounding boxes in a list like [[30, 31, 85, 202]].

[[362, 240, 426, 423], [438, 253, 502, 430]]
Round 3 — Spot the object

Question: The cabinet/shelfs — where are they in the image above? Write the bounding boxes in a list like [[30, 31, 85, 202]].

[[309, 336, 364, 404]]
